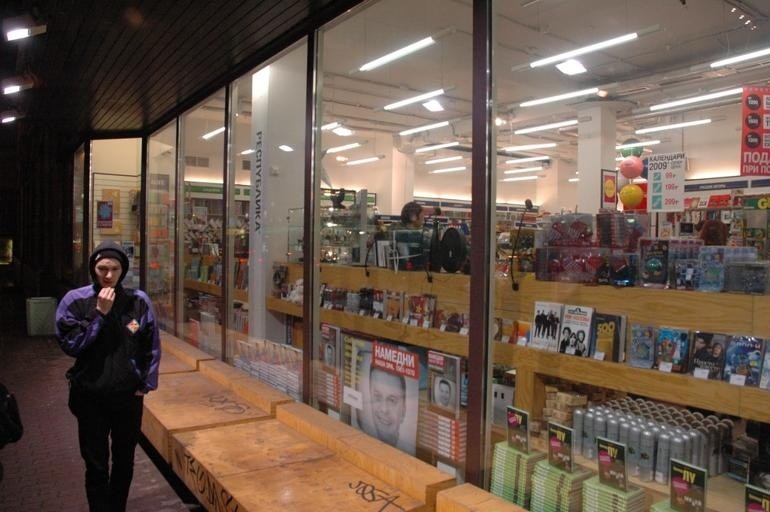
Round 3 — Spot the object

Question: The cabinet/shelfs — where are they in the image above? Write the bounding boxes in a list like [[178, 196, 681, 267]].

[[180, 252, 248, 346], [530, 279, 770, 512], [267, 261, 533, 444]]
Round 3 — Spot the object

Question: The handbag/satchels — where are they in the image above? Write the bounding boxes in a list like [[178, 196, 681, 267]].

[[0, 390, 23, 447]]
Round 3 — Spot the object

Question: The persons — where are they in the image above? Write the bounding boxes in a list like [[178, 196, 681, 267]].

[[691, 333, 715, 370], [707, 342, 725, 381], [326, 344, 335, 366], [698, 218, 729, 250], [54, 240, 160, 511], [436, 377, 454, 411], [401, 202, 423, 228], [533, 307, 587, 358], [368, 362, 407, 446]]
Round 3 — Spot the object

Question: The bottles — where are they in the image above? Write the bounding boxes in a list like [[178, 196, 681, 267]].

[[352, 239, 360, 263], [429, 219, 441, 272], [571, 395, 733, 487], [375, 219, 390, 268]]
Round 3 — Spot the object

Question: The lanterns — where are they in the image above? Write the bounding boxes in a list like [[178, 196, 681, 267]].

[[615, 138, 654, 210]]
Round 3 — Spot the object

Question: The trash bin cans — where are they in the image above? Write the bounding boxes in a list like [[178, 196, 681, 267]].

[[26, 297, 57, 336]]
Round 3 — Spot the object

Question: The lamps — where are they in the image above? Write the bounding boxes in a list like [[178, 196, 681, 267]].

[[0, 18, 45, 126]]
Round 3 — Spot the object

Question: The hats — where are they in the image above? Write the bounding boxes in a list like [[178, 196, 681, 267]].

[[93, 250, 124, 269]]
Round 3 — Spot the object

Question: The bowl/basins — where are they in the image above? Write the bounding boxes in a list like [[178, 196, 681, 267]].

[[440, 227, 466, 273]]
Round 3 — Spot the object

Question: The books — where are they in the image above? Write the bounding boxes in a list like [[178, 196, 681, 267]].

[[495, 297, 770, 392], [311, 281, 471, 485], [504, 404, 531, 456], [186, 253, 303, 402], [743, 485, 770, 512], [490, 436, 710, 512]]
